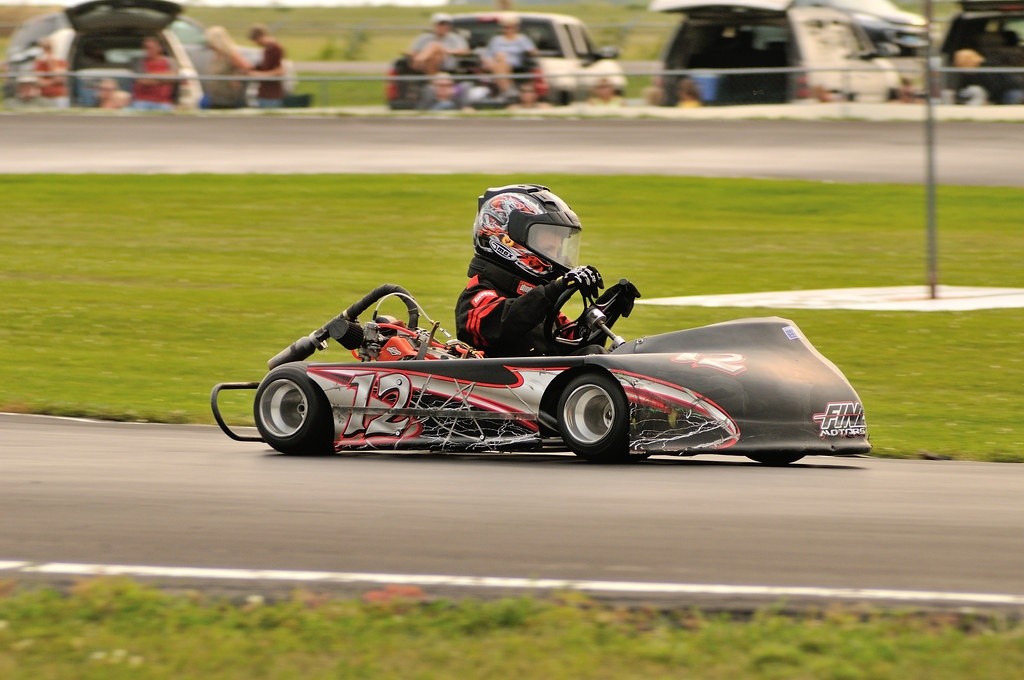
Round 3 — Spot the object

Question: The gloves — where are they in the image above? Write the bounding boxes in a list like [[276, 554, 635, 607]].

[[562, 265, 604, 299], [599, 280, 641, 318]]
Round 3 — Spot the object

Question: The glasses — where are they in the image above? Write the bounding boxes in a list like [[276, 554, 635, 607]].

[[436, 22, 451, 26]]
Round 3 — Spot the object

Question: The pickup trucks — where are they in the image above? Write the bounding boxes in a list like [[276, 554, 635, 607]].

[[392, 11, 627, 117]]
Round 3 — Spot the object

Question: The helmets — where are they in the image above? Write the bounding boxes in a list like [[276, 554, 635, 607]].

[[468, 184, 582, 298]]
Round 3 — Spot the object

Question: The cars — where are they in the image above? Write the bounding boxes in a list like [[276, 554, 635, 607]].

[[4, 0, 294, 108], [924, 1, 1024, 110], [641, 2, 938, 107]]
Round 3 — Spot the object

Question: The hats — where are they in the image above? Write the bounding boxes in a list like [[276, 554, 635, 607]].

[[430, 13, 452, 24]]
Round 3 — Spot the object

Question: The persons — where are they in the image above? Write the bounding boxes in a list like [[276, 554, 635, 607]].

[[10, 12, 703, 110], [455, 182, 644, 356]]
